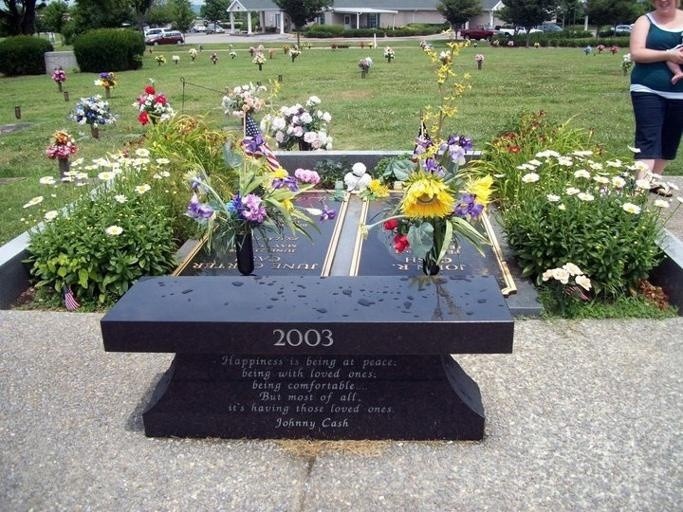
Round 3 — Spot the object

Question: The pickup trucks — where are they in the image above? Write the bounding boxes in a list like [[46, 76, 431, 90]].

[[499, 23, 545, 38], [460, 24, 502, 41]]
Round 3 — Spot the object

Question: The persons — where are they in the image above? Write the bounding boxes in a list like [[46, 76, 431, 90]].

[[628, 0, 683, 197], [666, 46, 683, 86]]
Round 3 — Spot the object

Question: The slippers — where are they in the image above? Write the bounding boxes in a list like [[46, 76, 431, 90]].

[[649, 184, 672, 197]]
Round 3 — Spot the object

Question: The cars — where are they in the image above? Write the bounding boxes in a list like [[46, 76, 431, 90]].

[[144, 23, 225, 45], [610, 23, 632, 33], [531, 22, 563, 34]]
